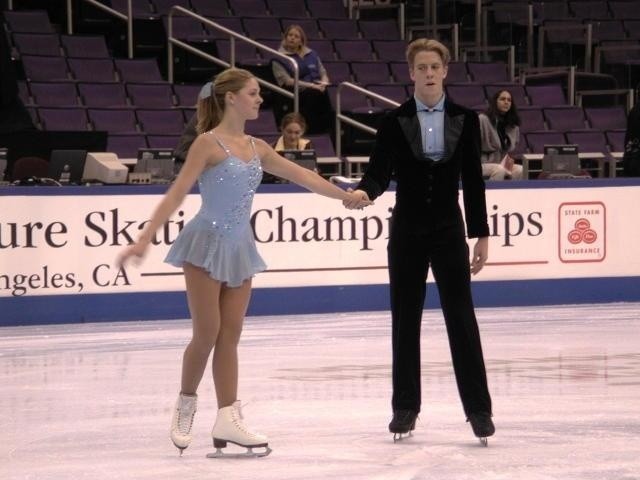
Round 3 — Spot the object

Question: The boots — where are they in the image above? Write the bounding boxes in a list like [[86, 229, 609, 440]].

[[212, 400, 268, 448], [170, 392, 198, 449], [389, 409, 418, 433], [466, 410, 495, 437]]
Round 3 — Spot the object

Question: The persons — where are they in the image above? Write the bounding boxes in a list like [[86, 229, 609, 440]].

[[262, 112, 321, 183], [342, 39, 496, 447], [624, 100, 640, 177], [478, 89, 525, 182], [114, 68, 375, 459], [271, 24, 334, 133]]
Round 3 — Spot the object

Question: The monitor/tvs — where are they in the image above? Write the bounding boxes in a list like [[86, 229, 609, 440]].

[[543, 144, 579, 158], [137, 148, 174, 161], [80, 150, 130, 185], [261, 149, 318, 183]]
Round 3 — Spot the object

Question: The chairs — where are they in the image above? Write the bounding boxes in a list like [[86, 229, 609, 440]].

[[0, 0, 640, 181]]
